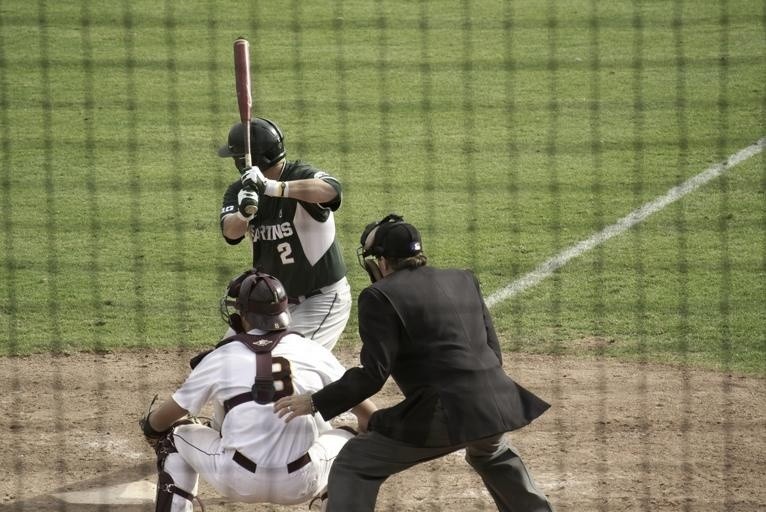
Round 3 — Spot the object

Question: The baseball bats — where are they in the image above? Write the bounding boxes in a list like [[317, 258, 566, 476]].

[[234, 36, 259, 215]]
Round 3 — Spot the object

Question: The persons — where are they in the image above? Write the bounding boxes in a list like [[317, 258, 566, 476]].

[[141, 272, 377, 512], [274, 216, 556, 512], [218, 117, 352, 352]]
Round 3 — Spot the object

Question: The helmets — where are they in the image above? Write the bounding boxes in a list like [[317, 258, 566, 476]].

[[356, 214, 423, 283], [219, 268, 289, 335], [218, 117, 287, 171]]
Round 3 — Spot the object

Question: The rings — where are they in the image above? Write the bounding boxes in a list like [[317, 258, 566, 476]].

[[287, 405, 290, 412]]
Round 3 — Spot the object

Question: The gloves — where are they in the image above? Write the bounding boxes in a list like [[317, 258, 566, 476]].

[[241, 166, 267, 195], [236, 185, 258, 218]]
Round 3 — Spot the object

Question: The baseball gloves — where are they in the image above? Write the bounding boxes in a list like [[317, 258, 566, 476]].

[[139, 394, 212, 457]]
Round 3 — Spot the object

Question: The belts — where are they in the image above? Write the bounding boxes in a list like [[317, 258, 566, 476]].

[[231, 449, 311, 474], [287, 289, 321, 305]]
